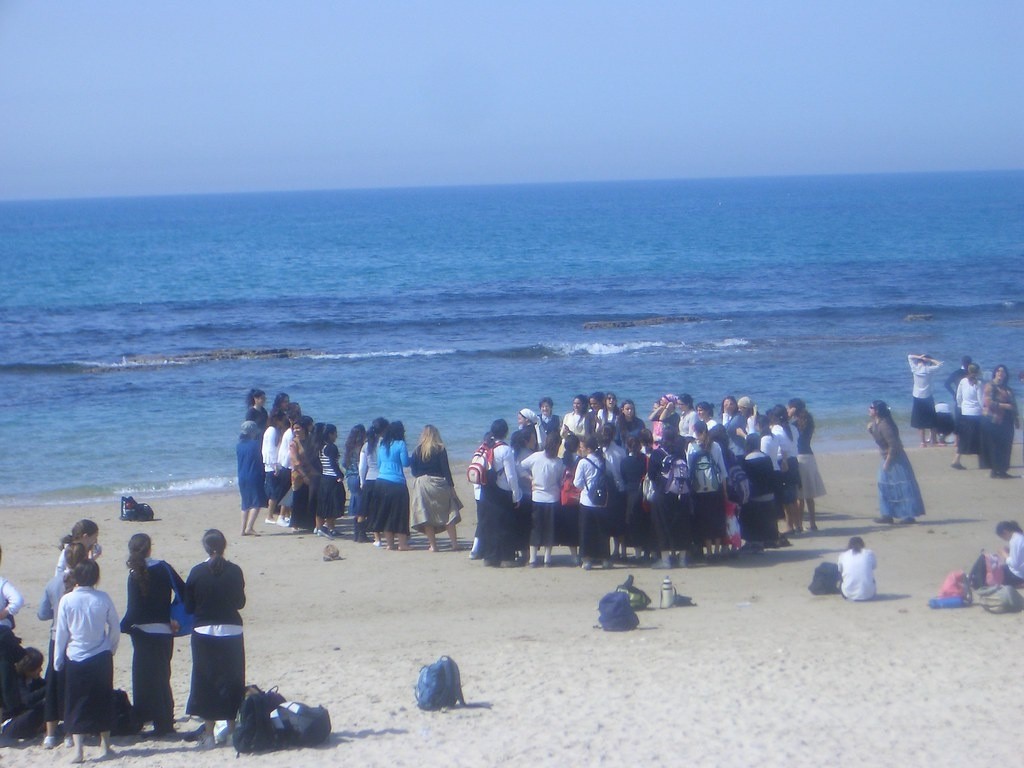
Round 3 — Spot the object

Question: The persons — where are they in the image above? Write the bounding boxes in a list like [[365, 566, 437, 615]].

[[119, 534, 186, 736], [996, 521, 1024, 584], [55, 519, 102, 574], [841, 536, 877, 601], [38, 543, 86, 745], [908, 353, 944, 446], [186, 527, 246, 747], [952, 364, 984, 470], [945, 356, 972, 446], [867, 400, 926, 524], [471, 392, 827, 566], [0, 549, 23, 630], [0, 636, 45, 747], [982, 365, 1021, 479], [53, 560, 117, 761], [927, 402, 954, 443], [15, 647, 46, 707], [237, 387, 463, 550]]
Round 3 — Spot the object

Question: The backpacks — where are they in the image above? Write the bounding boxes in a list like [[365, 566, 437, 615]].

[[416, 655, 466, 711], [617, 575, 651, 610], [268, 698, 332, 748], [979, 583, 1024, 615], [939, 570, 975, 607], [969, 550, 1005, 589], [808, 562, 842, 596], [232, 687, 285, 755], [109, 690, 144, 736], [597, 586, 638, 632], [120, 496, 138, 521], [466, 439, 750, 506], [137, 504, 154, 520]]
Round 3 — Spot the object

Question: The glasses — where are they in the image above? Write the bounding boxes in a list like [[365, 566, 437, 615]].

[[869, 405, 875, 410]]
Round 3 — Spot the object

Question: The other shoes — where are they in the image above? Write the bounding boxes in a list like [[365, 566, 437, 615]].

[[899, 516, 918, 524], [875, 516, 894, 523], [40, 719, 233, 766], [245, 515, 818, 568], [921, 434, 1010, 476]]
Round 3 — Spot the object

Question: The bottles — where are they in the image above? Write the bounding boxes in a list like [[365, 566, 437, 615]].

[[927, 597, 963, 608], [661, 576, 676, 608]]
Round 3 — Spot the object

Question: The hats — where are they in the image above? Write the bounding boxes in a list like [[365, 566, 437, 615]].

[[738, 396, 755, 408], [520, 408, 540, 424], [240, 421, 262, 436], [661, 394, 679, 408]]
